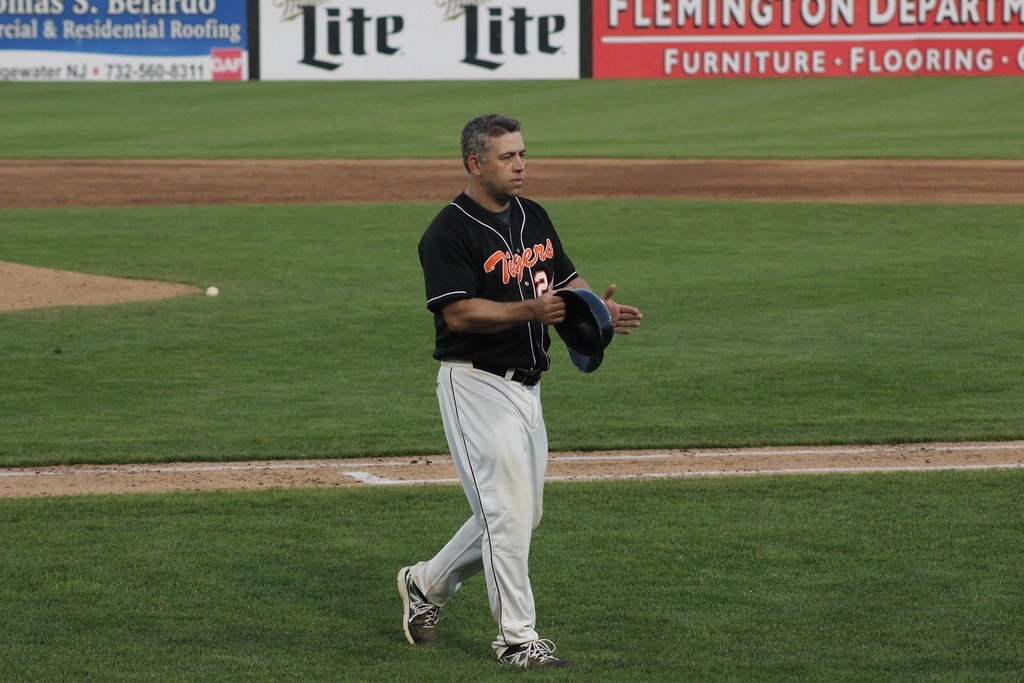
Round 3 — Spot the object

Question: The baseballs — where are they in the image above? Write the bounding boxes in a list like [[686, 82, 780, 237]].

[[205, 286, 219, 297]]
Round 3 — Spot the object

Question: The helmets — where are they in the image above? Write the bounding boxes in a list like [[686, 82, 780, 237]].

[[550, 289, 613, 374]]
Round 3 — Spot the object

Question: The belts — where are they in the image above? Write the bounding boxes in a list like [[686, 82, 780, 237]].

[[472, 361, 542, 386]]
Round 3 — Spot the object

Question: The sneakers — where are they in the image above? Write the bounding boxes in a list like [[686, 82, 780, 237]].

[[397, 566, 442, 646], [498, 638, 570, 671]]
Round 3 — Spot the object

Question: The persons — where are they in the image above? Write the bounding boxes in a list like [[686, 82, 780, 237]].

[[396, 113, 644, 670]]
